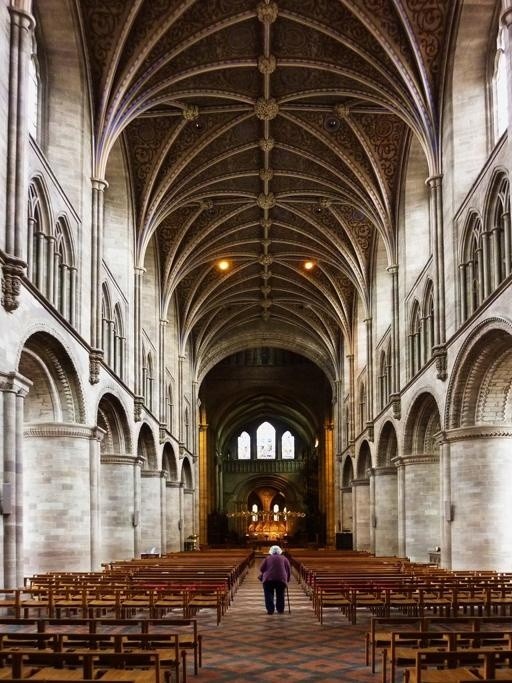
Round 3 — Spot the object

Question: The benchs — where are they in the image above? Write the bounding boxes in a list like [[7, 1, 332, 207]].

[[286, 545, 512, 683], [0, 540, 255, 683]]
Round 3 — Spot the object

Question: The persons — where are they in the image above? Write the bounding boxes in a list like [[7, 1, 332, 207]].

[[258, 544, 292, 615]]
